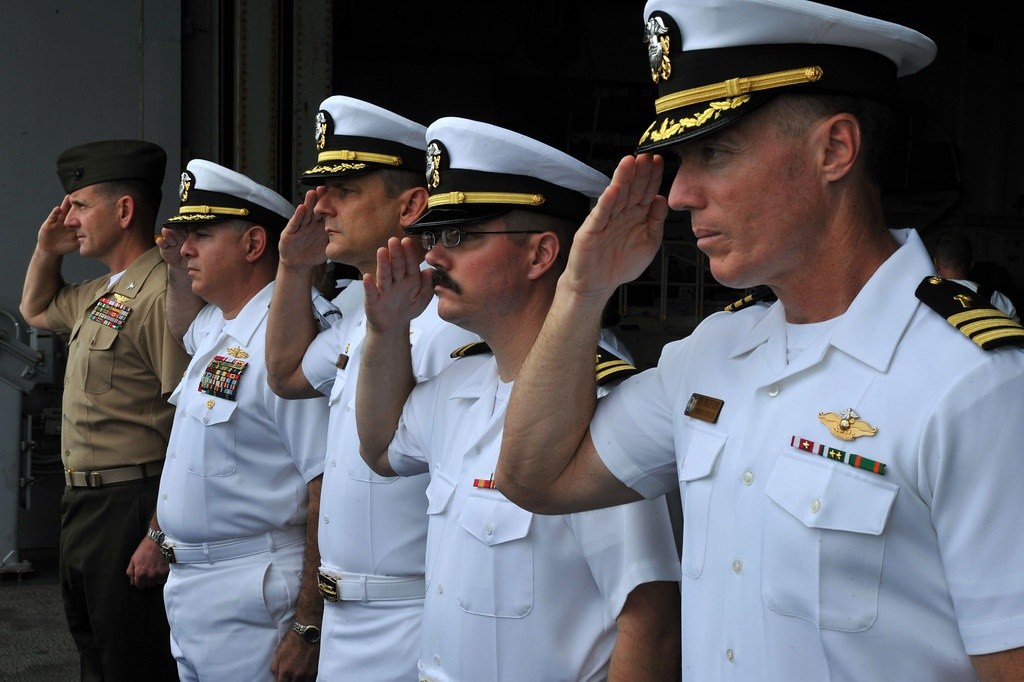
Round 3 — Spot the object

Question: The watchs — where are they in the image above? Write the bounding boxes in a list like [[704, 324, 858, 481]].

[[147, 527, 165, 545], [291, 619, 321, 642]]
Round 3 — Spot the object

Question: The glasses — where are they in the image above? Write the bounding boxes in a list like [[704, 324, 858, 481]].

[[421, 226, 561, 259]]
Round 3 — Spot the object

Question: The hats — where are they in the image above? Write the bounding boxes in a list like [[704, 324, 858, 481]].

[[297, 95, 428, 188], [56, 139, 167, 195], [632, 0, 938, 156], [404, 117, 612, 236], [163, 159, 296, 234]]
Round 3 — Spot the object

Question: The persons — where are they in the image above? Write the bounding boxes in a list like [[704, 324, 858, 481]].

[[265, 96, 483, 682], [496, 0, 1024, 682], [933, 233, 1020, 323], [156, 158, 331, 682], [19, 139, 192, 682], [356, 117, 682, 682]]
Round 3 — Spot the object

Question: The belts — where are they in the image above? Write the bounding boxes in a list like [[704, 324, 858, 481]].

[[64, 460, 165, 487], [160, 525, 306, 564], [316, 572, 426, 604]]
